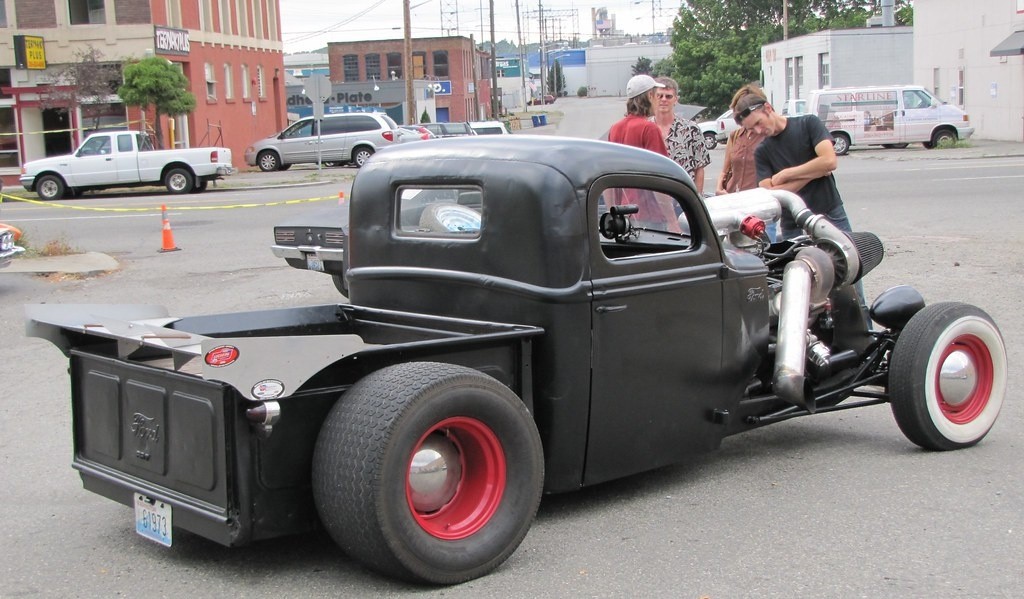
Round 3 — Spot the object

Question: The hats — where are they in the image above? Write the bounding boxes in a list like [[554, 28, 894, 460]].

[[627, 75, 666, 99]]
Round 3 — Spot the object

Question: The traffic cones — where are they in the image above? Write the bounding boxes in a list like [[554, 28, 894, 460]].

[[156, 204, 182, 253]]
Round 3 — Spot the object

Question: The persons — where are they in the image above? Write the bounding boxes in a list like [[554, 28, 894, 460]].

[[734, 93, 874, 331], [607, 75, 685, 233], [715, 86, 777, 243], [647, 77, 710, 217], [864, 110, 873, 131]]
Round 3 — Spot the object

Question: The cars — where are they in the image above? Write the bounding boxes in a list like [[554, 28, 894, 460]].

[[22, 133, 1010, 584], [397, 121, 508, 145], [528, 95, 555, 105], [696, 109, 743, 150]]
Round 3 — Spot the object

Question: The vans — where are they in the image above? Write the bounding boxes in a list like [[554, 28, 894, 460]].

[[809, 85, 974, 156], [244, 112, 403, 171]]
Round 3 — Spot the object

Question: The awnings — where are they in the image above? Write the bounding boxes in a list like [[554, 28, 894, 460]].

[[990, 31, 1024, 57]]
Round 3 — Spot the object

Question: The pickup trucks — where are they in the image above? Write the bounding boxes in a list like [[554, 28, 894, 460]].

[[19, 130, 232, 201]]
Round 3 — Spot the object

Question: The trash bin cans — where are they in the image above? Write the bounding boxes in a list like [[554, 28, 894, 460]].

[[539, 114, 547, 126], [532, 115, 540, 127]]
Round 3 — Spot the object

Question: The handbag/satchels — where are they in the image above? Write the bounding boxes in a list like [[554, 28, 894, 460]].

[[722, 129, 740, 190]]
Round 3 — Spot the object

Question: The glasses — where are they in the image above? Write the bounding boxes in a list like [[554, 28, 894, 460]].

[[657, 93, 673, 99], [734, 103, 763, 121]]
